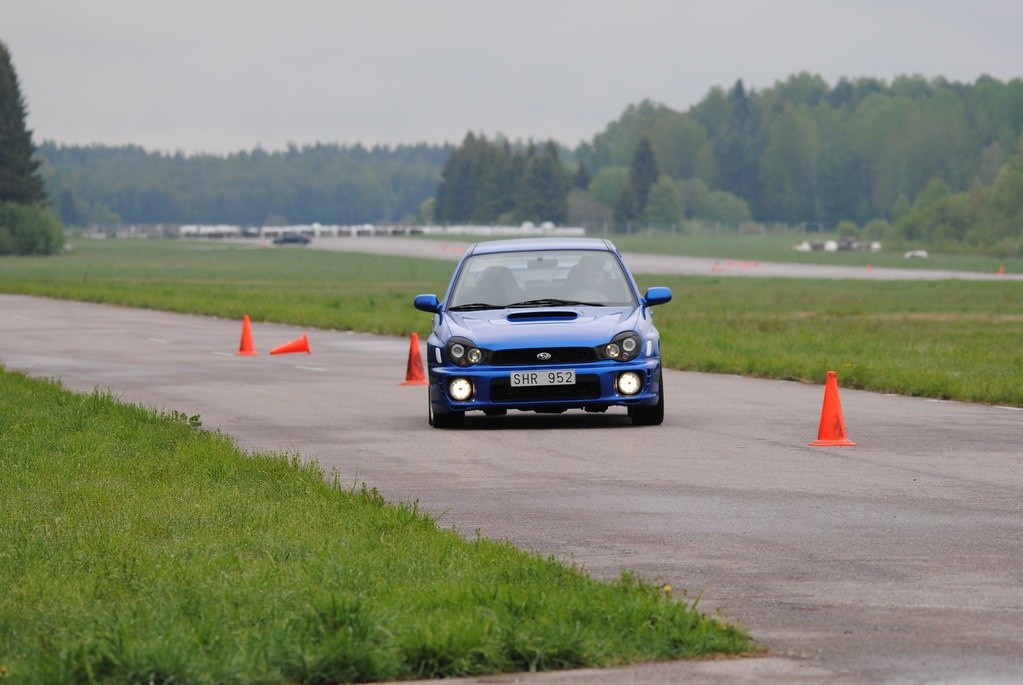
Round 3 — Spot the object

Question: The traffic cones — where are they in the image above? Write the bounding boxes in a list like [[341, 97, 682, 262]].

[[270, 334, 312, 355], [807, 371, 857, 446], [234, 314, 259, 355], [400, 331, 429, 386]]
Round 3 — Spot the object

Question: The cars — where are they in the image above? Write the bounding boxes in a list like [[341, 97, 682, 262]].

[[413, 236, 673, 427], [273, 233, 310, 245]]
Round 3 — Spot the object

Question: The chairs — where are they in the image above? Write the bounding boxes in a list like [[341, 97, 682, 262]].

[[474, 266, 518, 303]]
